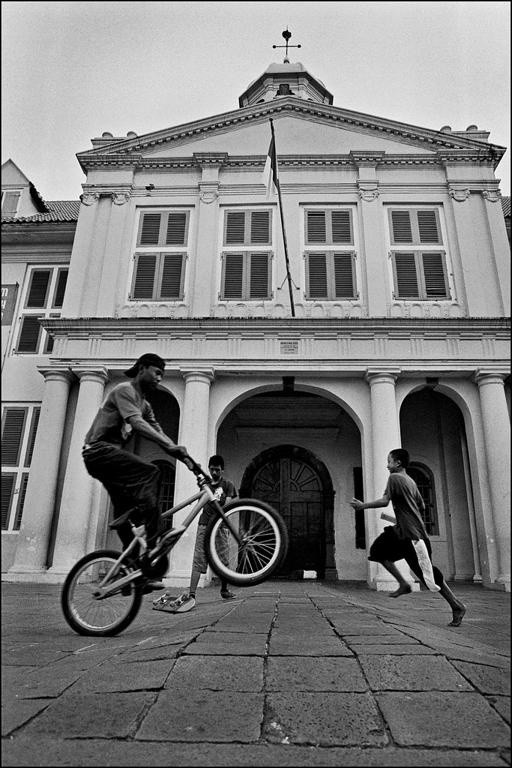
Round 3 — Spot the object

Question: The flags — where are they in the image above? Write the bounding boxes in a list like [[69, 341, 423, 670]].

[[263, 136, 279, 197]]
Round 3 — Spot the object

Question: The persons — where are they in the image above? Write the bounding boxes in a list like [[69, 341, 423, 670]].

[[83, 354, 187, 594], [349, 448, 467, 627], [183, 454, 241, 601]]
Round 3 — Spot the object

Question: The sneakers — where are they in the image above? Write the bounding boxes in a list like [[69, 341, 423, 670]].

[[220, 591, 235, 598], [146, 526, 186, 558], [122, 588, 152, 596]]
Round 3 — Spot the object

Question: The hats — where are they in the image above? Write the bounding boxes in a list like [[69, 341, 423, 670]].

[[124, 354, 166, 378]]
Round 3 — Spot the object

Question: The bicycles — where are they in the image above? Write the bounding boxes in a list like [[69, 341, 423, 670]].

[[62, 446, 289, 637]]
